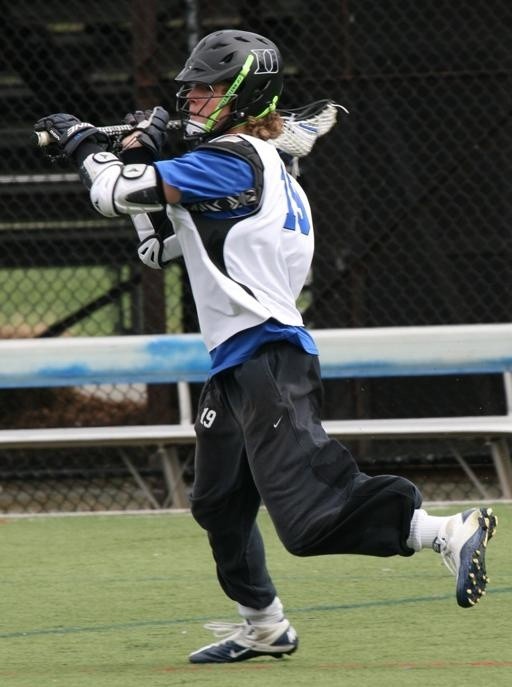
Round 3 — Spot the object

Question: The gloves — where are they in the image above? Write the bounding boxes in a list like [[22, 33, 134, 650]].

[[116, 104, 171, 165], [32, 113, 111, 167]]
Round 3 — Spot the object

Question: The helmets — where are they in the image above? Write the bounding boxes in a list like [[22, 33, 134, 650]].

[[176, 30, 284, 145]]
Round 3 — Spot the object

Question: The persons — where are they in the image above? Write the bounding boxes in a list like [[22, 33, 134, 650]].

[[32, 30, 498, 663]]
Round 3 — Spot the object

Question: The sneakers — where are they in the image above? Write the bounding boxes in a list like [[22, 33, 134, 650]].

[[187, 617, 299, 665], [432, 506, 498, 609]]
